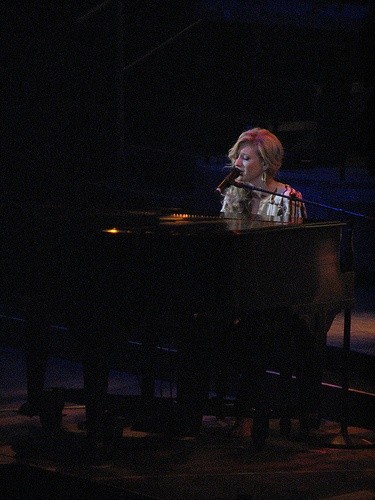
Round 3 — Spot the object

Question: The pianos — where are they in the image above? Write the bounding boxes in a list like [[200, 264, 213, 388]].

[[24, 203, 353, 426]]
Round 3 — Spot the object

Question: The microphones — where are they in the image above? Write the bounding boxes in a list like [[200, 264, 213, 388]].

[[216, 167, 240, 194]]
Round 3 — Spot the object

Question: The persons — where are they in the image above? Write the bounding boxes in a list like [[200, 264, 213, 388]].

[[216, 128, 309, 437]]
